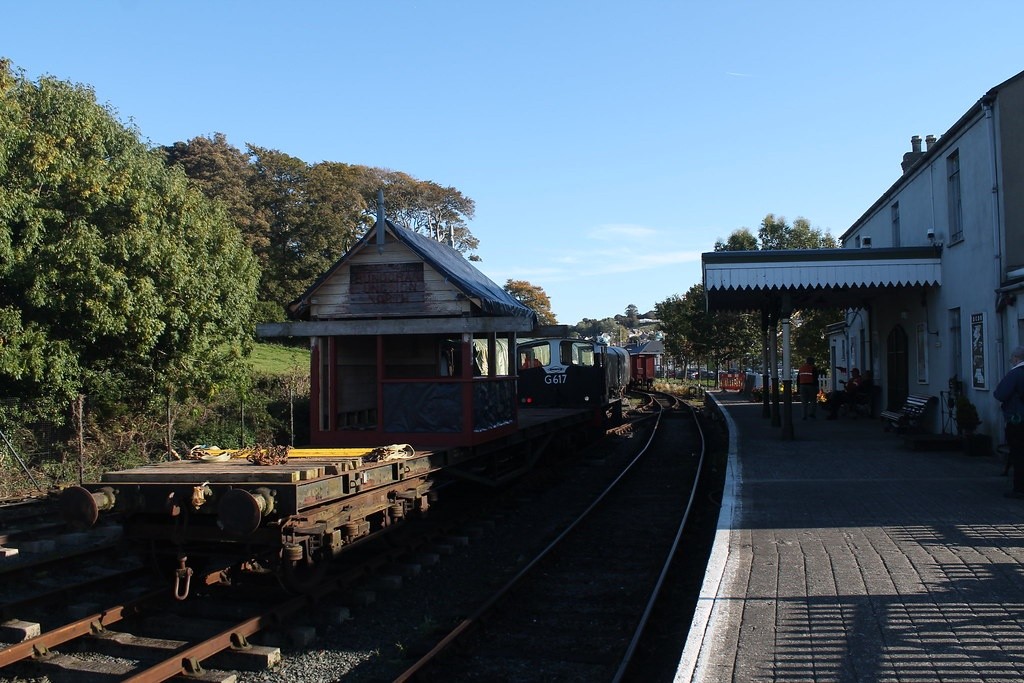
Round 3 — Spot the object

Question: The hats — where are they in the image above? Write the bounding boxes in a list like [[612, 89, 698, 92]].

[[849, 368, 859, 373]]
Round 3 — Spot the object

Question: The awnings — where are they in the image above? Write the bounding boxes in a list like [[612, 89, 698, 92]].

[[703, 246, 944, 313]]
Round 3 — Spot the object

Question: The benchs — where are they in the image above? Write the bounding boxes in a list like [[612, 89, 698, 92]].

[[880, 392, 939, 437]]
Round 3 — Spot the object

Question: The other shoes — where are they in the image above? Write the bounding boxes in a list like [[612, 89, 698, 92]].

[[802, 416, 807, 420], [808, 414, 816, 419], [827, 413, 838, 420], [1003, 491, 1024, 499]]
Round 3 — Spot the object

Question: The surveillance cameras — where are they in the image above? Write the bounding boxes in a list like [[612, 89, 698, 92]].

[[927, 229, 934, 239]]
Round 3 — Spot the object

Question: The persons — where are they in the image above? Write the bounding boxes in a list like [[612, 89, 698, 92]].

[[797, 357, 819, 420], [838, 368, 862, 403], [993, 345, 1024, 500]]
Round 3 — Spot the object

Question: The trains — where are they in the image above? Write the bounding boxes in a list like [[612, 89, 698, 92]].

[[515, 338, 631, 421]]
